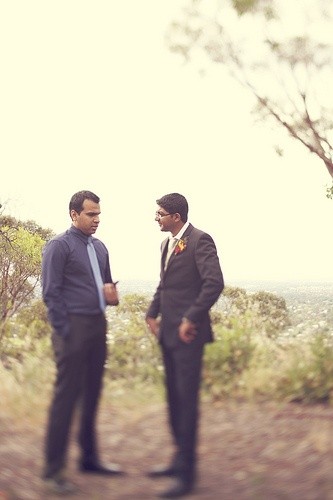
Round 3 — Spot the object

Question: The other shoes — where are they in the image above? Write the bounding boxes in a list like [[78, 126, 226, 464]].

[[41, 468, 74, 492], [161, 486, 191, 499], [148, 467, 176, 478], [78, 464, 123, 477]]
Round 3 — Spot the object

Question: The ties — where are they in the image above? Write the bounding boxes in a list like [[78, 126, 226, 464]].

[[87, 237, 105, 312], [164, 239, 176, 271]]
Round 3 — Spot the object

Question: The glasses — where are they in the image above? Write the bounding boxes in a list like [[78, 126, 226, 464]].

[[155, 211, 174, 218]]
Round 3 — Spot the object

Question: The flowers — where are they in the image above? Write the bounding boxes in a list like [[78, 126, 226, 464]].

[[171, 236, 189, 255]]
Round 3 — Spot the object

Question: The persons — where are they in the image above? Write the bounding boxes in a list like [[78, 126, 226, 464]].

[[43, 190, 123, 483], [146, 193, 225, 499]]
